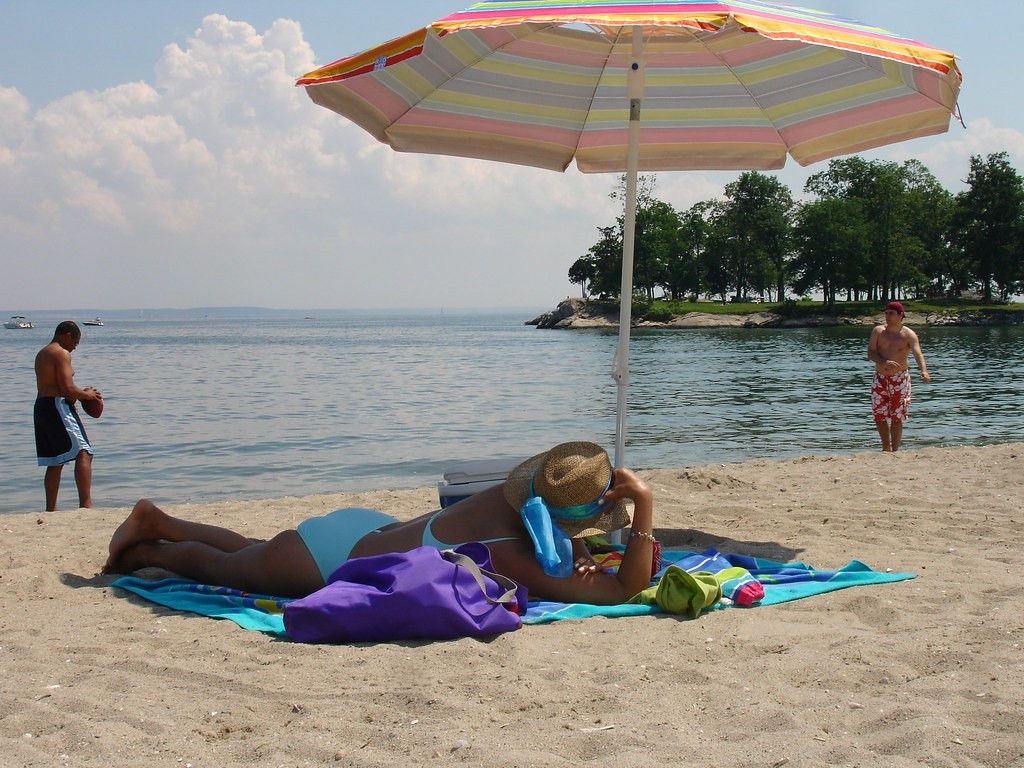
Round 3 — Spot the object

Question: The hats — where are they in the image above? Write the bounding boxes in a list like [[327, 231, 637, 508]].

[[504, 441, 636, 579], [886, 302, 906, 318]]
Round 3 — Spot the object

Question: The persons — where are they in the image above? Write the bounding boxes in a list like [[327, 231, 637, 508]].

[[103, 442, 654, 605], [33, 321, 103, 511], [868, 301, 929, 452]]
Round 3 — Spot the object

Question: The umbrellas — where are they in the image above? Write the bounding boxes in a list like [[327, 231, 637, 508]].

[[295, 0, 963, 548]]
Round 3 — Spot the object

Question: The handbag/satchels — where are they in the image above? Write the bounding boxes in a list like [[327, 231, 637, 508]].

[[283, 541, 529, 644]]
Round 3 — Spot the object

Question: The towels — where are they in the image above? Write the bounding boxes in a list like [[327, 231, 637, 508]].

[[107, 535, 920, 642]]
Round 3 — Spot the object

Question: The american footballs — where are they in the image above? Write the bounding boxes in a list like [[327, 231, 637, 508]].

[[80, 387, 104, 419]]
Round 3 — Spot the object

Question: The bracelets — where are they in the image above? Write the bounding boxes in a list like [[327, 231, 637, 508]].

[[629, 532, 655, 542]]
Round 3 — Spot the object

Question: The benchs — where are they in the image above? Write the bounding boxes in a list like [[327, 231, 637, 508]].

[[696, 299, 714, 304], [751, 299, 760, 304]]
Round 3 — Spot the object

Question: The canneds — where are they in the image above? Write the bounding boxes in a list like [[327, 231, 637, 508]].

[[651, 541, 661, 575]]
